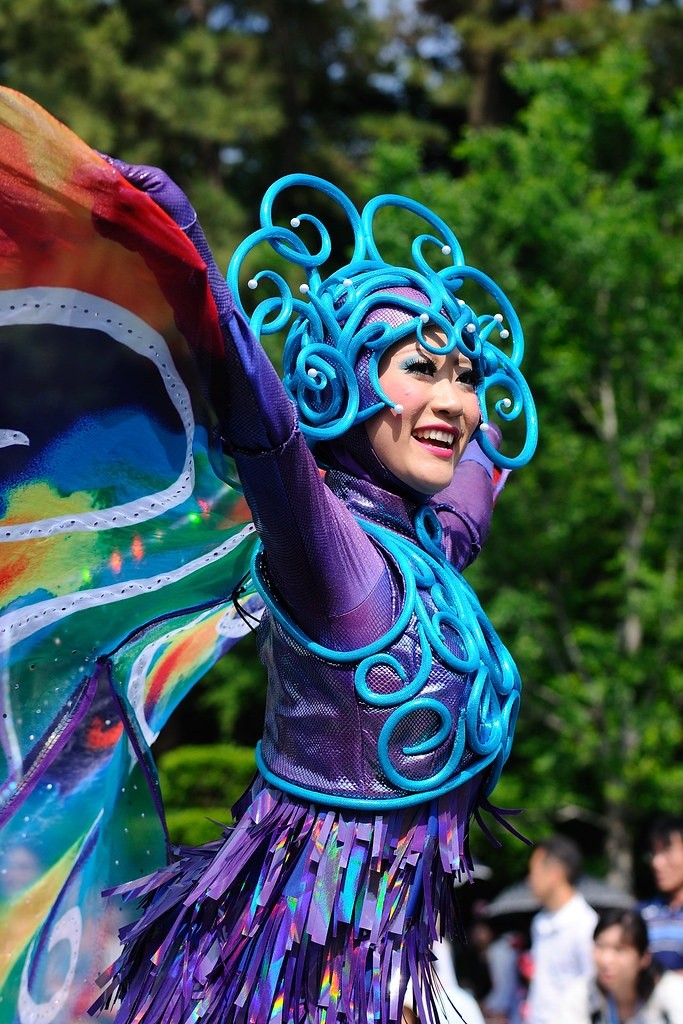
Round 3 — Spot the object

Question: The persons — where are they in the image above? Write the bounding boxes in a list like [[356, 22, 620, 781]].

[[427, 816, 683, 1023], [0, 842, 161, 1024], [0, 87, 542, 1024]]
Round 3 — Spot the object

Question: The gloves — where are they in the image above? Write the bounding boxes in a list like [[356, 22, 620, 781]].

[[74, 148, 234, 333], [459, 421, 503, 479]]
